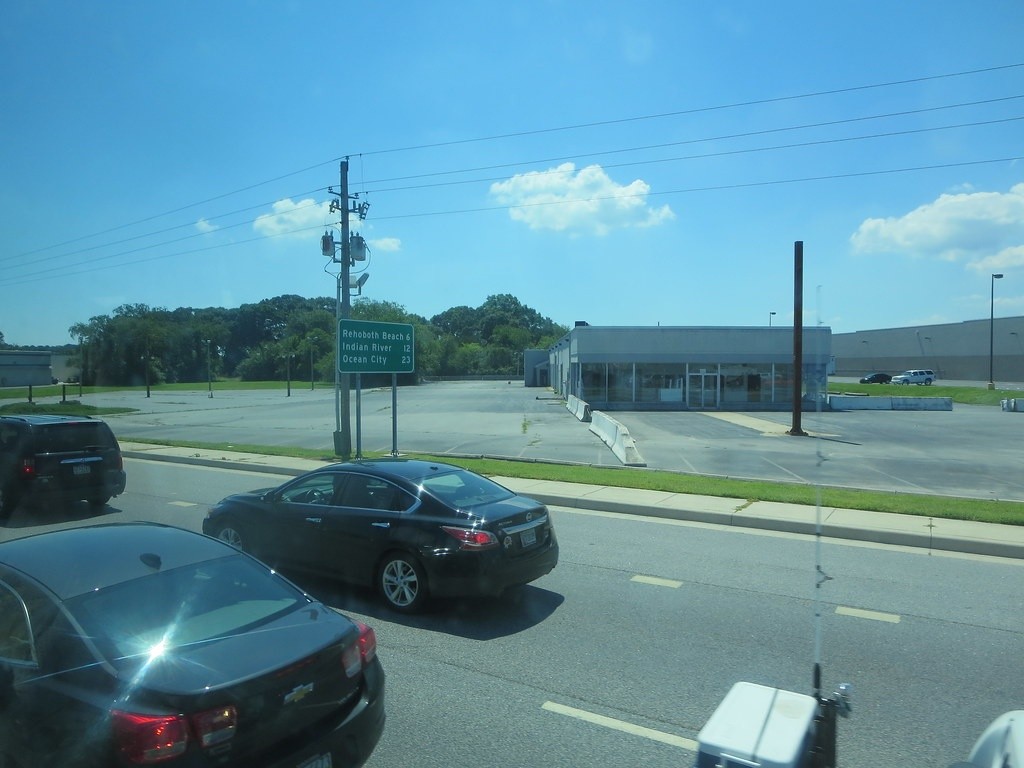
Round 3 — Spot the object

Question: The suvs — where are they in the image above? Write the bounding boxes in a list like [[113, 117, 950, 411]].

[[0, 413, 126, 521], [891, 369, 936, 385]]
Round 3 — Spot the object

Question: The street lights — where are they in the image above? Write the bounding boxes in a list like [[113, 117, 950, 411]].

[[201, 340, 214, 398], [986, 271, 1004, 390], [307, 336, 319, 390]]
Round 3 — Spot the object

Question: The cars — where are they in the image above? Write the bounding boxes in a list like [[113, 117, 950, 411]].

[[860, 373, 892, 384], [202, 457, 559, 615], [0, 521, 386, 768]]
